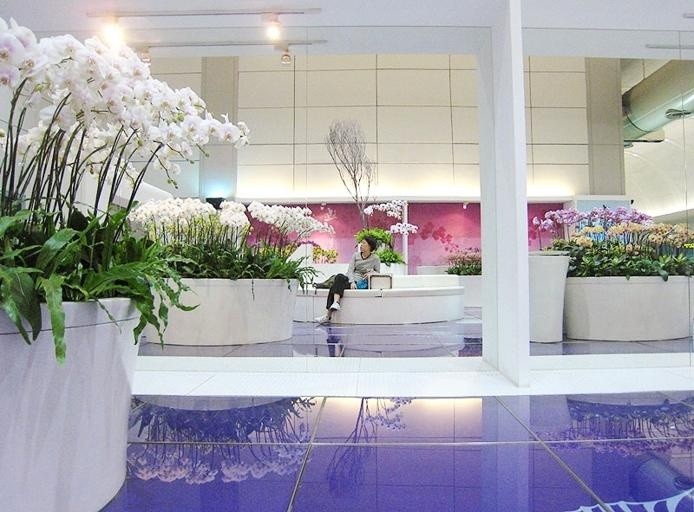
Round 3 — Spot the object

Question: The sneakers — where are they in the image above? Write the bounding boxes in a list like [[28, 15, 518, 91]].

[[314, 314, 333, 324], [329, 301, 340, 312]]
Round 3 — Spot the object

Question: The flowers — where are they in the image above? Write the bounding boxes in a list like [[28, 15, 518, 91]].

[[124, 396, 316, 486], [363, 198, 419, 235], [530, 205, 694, 281], [0, 17, 250, 363], [534, 401, 694, 455], [124, 196, 335, 295]]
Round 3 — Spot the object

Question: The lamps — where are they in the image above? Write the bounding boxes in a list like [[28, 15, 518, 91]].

[[99, 11, 305, 41], [130, 42, 312, 68]]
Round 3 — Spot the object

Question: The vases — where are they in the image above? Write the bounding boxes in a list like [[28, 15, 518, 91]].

[[564, 274, 693, 341], [135, 396, 284, 412], [529, 256, 571, 343], [0, 295, 139, 512], [564, 391, 694, 406], [138, 278, 300, 345]]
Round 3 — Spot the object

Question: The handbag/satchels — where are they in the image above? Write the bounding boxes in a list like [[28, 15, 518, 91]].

[[312, 272, 337, 289]]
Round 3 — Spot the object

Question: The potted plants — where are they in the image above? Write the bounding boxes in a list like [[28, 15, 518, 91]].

[[445, 247, 482, 308], [354, 226, 393, 252], [375, 249, 405, 275]]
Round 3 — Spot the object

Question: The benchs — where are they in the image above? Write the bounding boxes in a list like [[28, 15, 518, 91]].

[[294, 286, 466, 325]]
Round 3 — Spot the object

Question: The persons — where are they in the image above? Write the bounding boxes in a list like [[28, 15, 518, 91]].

[[314, 234, 381, 325]]
